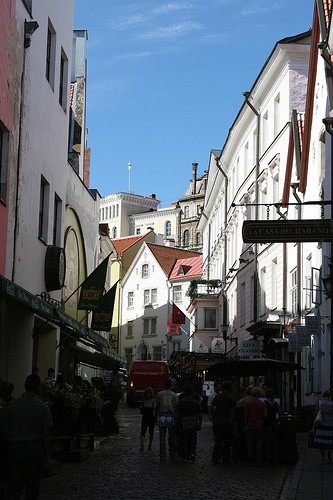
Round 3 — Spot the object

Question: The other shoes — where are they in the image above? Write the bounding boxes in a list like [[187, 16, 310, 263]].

[[148, 446, 152, 450], [184, 458, 189, 462], [160, 457, 166, 462], [213, 459, 223, 463], [171, 456, 175, 460], [255, 463, 264, 466], [140, 447, 144, 451]]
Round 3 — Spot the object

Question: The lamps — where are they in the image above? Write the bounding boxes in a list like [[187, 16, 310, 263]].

[[247, 250, 254, 256], [220, 322, 238, 345], [229, 268, 239, 273], [225, 276, 233, 280], [238, 258, 250, 264]]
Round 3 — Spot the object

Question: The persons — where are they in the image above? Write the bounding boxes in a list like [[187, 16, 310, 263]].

[[154, 379, 181, 463], [1, 374, 53, 500], [33, 366, 65, 387], [140, 387, 156, 451], [206, 380, 269, 464], [311, 389, 333, 464], [175, 386, 203, 460]]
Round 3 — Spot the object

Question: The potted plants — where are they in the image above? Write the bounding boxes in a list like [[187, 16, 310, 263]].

[[42, 383, 105, 457]]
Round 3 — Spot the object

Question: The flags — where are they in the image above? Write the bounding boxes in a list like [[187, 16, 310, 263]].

[[166, 303, 180, 334], [91, 283, 117, 332], [76, 256, 109, 312], [172, 304, 186, 324]]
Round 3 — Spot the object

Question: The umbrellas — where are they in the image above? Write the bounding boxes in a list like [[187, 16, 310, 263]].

[[203, 357, 305, 395]]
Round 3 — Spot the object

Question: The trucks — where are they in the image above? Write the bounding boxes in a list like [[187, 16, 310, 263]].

[[126, 360, 169, 408]]
